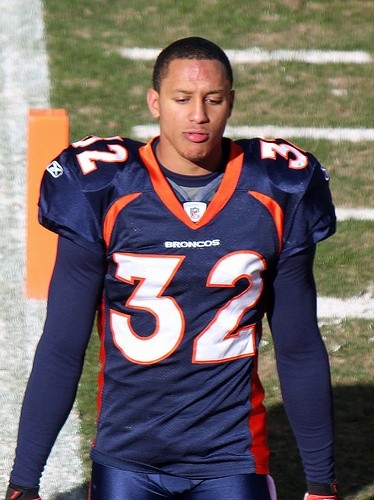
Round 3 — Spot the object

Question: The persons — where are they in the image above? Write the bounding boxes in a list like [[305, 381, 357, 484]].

[[8, 37, 338, 500]]
